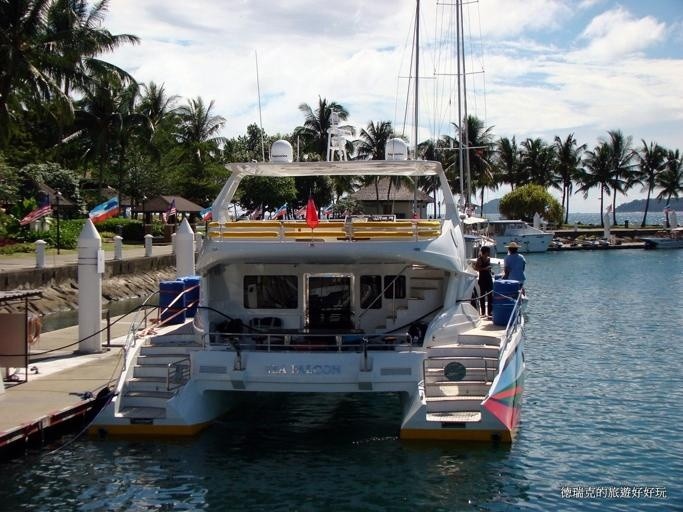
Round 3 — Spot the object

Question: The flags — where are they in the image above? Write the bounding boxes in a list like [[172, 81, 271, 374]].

[[162, 199, 176, 223], [293, 204, 307, 218], [305, 198, 318, 228], [323, 204, 333, 214], [199, 204, 213, 221], [88, 195, 118, 223], [271, 202, 287, 220], [248, 203, 262, 220], [18, 193, 54, 226]]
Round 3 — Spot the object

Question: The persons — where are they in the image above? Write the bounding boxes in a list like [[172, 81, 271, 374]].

[[501, 242, 527, 281], [475, 245, 495, 318]]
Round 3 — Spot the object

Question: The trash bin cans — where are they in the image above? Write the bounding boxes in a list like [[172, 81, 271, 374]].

[[159, 275, 201, 325], [492, 278, 522, 326]]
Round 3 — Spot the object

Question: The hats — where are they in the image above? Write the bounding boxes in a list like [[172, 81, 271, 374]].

[[503, 242, 522, 248]]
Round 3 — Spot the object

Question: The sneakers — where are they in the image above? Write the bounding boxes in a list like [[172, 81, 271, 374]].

[[481, 314, 493, 319]]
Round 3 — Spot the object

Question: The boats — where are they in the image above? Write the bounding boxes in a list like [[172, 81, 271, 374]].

[[461, 217, 551, 258], [86, 161, 528, 446]]
